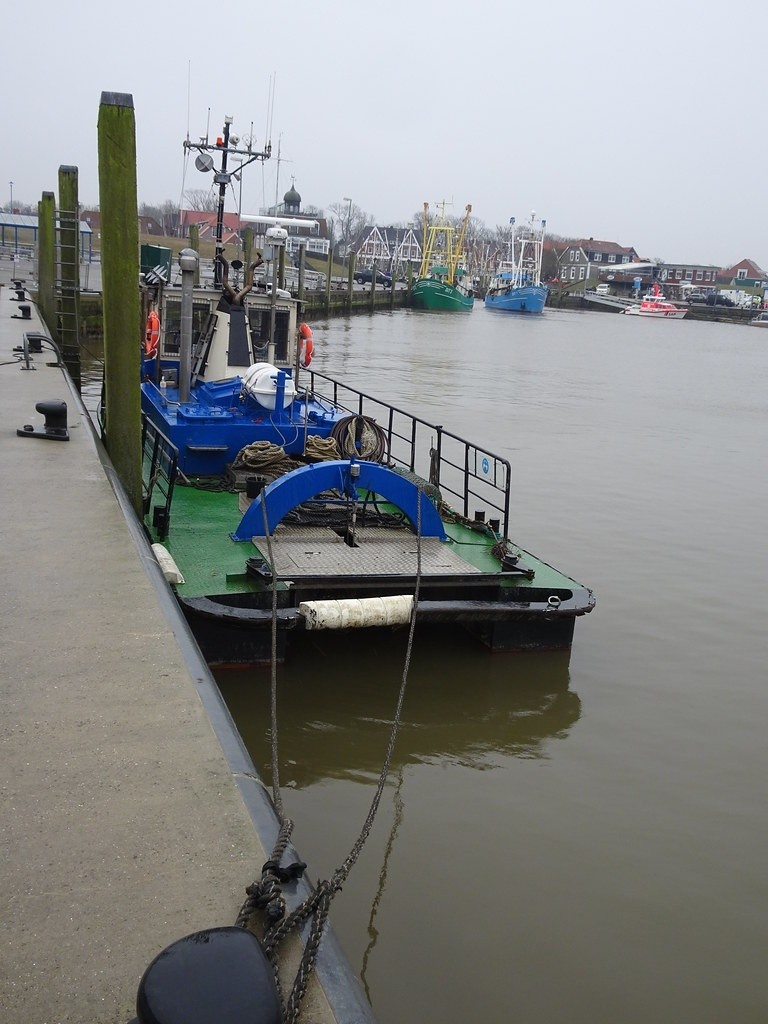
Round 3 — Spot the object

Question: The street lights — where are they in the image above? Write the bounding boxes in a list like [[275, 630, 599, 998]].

[[340, 197, 353, 290], [9, 180, 14, 215]]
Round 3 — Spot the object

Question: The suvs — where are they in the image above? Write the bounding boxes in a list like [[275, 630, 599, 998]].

[[706, 295, 735, 307], [685, 294, 706, 304]]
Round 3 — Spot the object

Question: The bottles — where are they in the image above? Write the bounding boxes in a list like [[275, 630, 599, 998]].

[[160, 376, 166, 396]]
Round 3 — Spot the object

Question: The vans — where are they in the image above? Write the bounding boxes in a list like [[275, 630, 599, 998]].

[[596, 284, 610, 295]]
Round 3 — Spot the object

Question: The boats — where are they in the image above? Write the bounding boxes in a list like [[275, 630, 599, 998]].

[[97, 60, 596, 658], [410, 194, 474, 311], [619, 280, 689, 319], [485, 209, 549, 313], [474, 240, 486, 299], [752, 313, 768, 324]]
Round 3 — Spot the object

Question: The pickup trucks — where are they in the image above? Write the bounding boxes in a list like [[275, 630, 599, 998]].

[[354, 269, 393, 287]]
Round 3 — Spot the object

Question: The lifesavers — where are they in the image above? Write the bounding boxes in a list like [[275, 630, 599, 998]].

[[298, 323, 312, 366], [144, 312, 160, 359]]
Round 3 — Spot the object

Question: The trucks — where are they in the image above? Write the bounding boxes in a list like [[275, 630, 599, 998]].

[[721, 290, 752, 305]]
